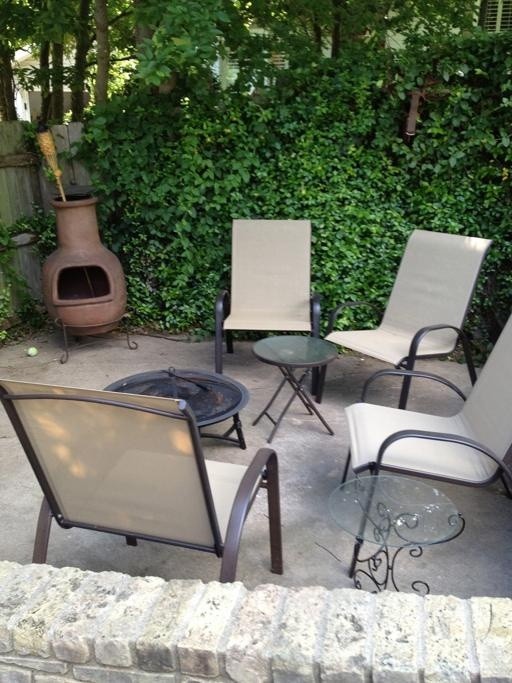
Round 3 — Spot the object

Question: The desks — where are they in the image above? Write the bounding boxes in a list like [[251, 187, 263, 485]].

[[328, 474, 466, 595], [251, 336, 338, 443]]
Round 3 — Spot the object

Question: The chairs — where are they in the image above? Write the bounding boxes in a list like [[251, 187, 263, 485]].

[[214, 219, 320, 396], [340, 314, 511, 579], [316, 228, 493, 411], [0, 378, 284, 584]]
